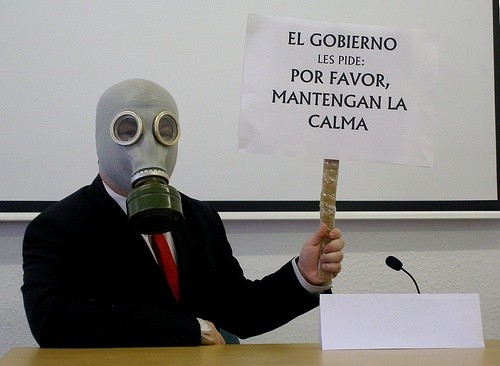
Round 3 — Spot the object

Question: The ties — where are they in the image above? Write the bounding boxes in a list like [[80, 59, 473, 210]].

[[150, 233, 182, 305]]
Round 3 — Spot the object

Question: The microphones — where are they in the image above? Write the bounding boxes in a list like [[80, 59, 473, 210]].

[[385, 255, 420, 294]]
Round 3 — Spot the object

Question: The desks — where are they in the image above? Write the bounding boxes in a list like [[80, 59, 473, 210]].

[[0, 340, 500, 366]]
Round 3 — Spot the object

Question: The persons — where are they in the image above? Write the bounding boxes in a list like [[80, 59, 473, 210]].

[[21, 78, 345, 347]]
